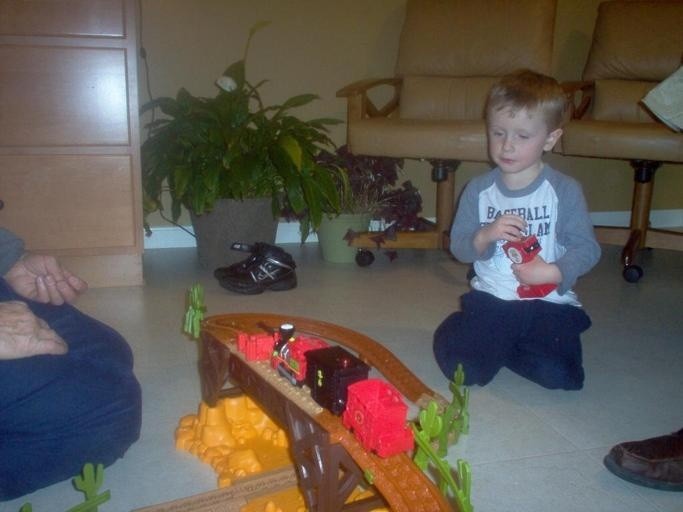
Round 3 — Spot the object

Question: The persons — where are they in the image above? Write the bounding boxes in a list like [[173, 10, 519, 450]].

[[0, 198, 141, 503], [430, 67, 604, 392]]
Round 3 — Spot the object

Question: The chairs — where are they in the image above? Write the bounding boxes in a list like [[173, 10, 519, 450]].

[[540, 0, 683, 285], [334, 0, 564, 275]]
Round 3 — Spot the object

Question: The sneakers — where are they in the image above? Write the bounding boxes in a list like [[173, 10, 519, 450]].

[[214, 242, 285, 282], [218, 247, 297, 295]]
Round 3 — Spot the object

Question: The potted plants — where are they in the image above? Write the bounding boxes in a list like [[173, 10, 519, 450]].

[[303, 147, 380, 265], [130, 13, 350, 276]]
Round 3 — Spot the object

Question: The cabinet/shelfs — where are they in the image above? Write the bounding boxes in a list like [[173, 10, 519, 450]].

[[0, 3, 149, 295]]
